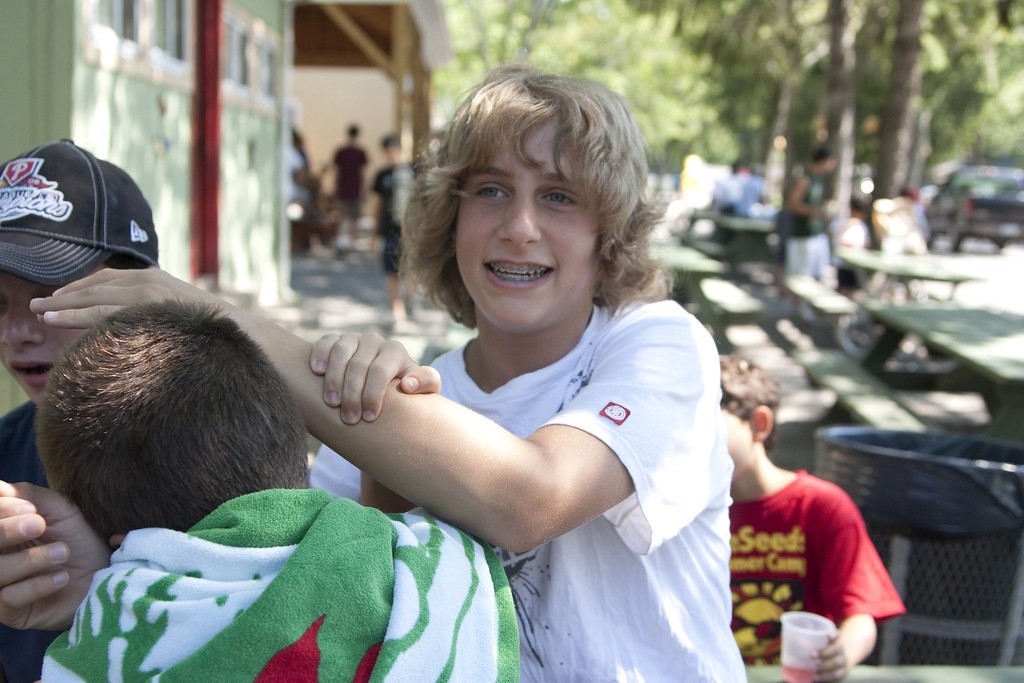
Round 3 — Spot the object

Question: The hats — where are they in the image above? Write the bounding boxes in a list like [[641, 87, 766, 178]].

[[0, 138, 160, 285]]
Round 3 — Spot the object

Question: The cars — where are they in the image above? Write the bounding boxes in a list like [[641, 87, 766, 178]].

[[920, 164, 1024, 253]]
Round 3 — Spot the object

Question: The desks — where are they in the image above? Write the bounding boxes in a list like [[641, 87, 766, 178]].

[[860, 297, 1023, 437], [830, 248, 988, 305]]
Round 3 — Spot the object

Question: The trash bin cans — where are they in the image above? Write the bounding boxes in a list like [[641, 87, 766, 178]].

[[812, 424, 1024, 668]]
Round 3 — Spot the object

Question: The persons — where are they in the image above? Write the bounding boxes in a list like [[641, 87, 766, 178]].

[[713, 148, 934, 306], [288, 123, 431, 336], [0, 64, 747, 683], [720, 353, 907, 683], [0, 139, 161, 683], [35, 293, 521, 683]]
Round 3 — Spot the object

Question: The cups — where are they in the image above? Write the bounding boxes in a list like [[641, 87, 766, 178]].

[[780, 612, 839, 683]]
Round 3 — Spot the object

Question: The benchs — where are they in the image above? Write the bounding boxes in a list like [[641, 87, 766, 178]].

[[654, 243, 925, 433]]
